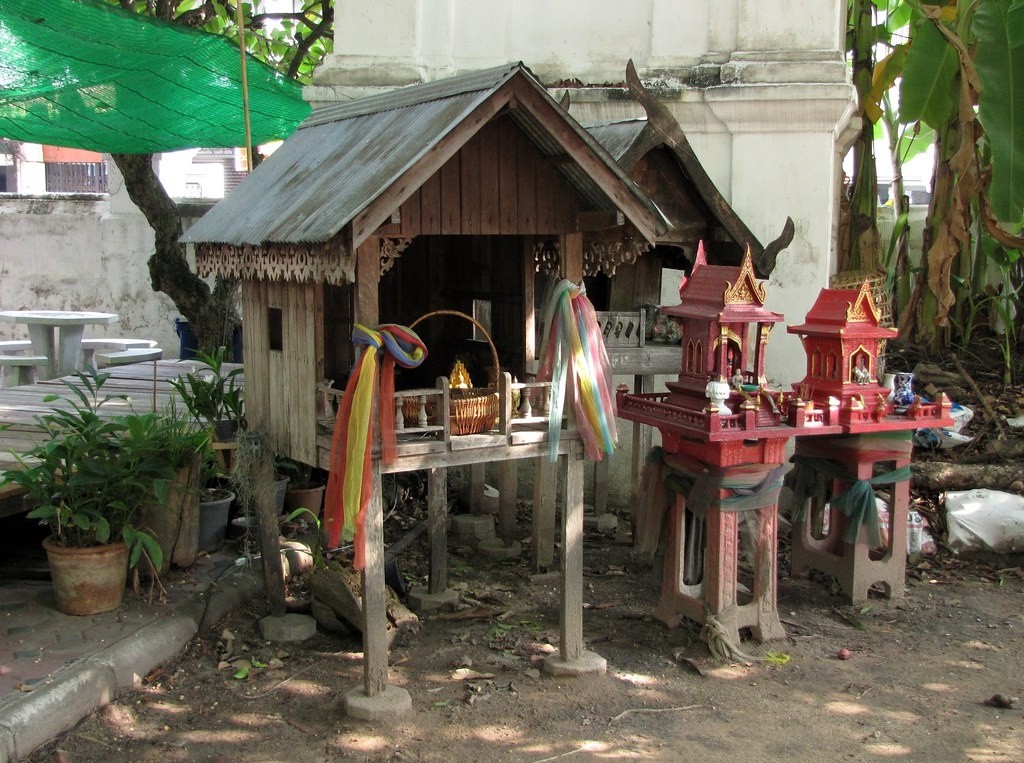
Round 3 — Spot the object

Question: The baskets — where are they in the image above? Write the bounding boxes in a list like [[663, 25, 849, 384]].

[[394, 310, 501, 435]]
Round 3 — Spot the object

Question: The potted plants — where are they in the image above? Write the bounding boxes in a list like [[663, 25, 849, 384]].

[[0, 345, 326, 615]]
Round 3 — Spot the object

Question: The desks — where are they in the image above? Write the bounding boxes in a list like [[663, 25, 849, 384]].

[[0, 310, 119, 381]]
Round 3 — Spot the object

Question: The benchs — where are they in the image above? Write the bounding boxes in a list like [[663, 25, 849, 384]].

[[0, 338, 163, 389]]
